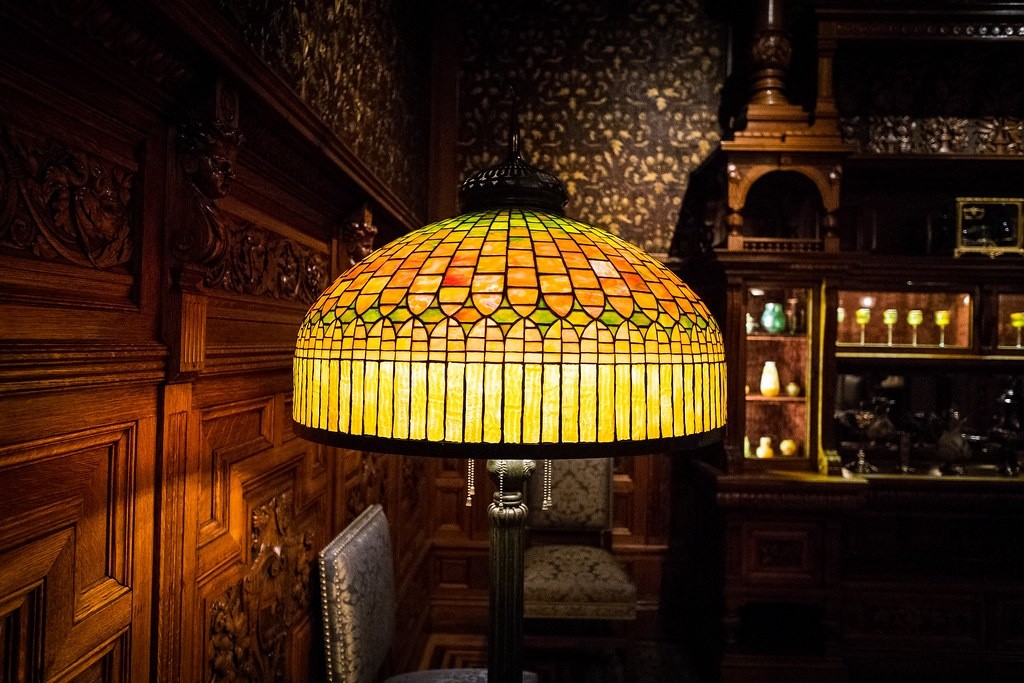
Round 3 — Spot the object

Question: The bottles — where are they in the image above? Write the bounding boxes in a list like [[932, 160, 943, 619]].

[[786, 299, 804, 335], [756, 437, 773, 458], [760, 361, 779, 396], [761, 302, 785, 334]]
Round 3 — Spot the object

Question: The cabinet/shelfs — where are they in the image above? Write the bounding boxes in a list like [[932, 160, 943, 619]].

[[722, 0, 1024, 683]]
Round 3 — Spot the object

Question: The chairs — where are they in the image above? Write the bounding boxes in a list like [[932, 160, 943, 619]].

[[316, 502, 542, 682], [520, 456, 637, 625]]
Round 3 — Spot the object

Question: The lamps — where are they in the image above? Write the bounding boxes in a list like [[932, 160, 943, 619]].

[[291, 101, 731, 683]]
